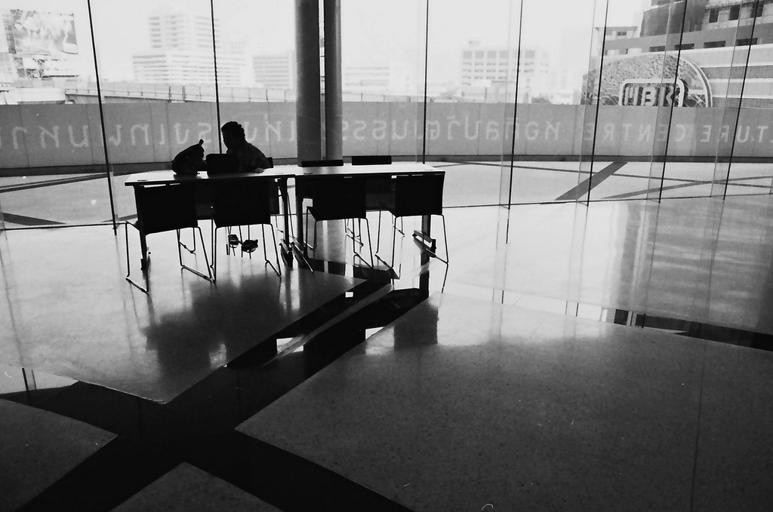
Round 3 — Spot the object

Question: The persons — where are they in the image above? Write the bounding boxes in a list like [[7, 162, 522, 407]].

[[221, 122, 270, 169]]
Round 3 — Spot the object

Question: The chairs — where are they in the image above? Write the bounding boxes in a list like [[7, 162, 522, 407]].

[[123, 153, 449, 292]]
